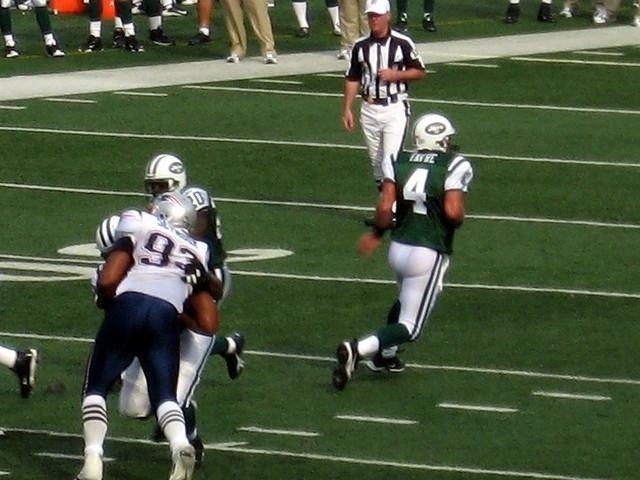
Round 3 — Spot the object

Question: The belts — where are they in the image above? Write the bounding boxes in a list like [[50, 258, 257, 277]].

[[364, 96, 408, 104]]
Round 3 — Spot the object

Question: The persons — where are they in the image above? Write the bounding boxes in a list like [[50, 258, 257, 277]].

[[332, 113, 475, 391], [341, 2, 426, 225], [109, 153, 245, 467], [75, 190, 221, 480], [0, 344, 37, 399]]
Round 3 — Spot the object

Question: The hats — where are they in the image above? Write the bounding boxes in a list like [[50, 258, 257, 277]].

[[362, 0, 391, 15]]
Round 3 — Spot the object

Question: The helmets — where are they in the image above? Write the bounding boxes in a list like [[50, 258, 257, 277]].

[[410, 112, 457, 153], [143, 153, 188, 193], [148, 193, 197, 232], [94, 214, 122, 256]]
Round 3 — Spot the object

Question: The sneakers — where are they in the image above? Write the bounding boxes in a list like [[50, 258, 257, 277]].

[[503, 5, 640, 27], [225, 47, 244, 63], [225, 331, 246, 381], [363, 352, 408, 374], [391, 15, 439, 33], [150, 420, 209, 480], [73, 460, 104, 480], [12, 348, 38, 399], [5, 40, 21, 58], [77, 27, 213, 54], [264, 48, 279, 65], [332, 339, 357, 391], [44, 40, 66, 58], [294, 25, 349, 60], [362, 211, 398, 230]]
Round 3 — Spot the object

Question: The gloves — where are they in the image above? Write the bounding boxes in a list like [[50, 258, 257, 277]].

[[180, 258, 209, 288]]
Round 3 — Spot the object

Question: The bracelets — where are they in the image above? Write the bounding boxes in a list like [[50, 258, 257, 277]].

[[373, 229, 384, 236]]
